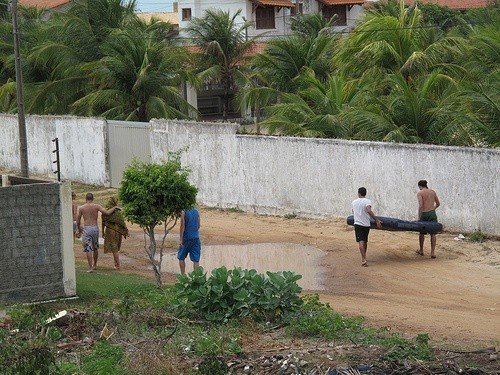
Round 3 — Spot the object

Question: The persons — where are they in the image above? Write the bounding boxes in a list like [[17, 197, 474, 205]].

[[177, 205, 201, 275], [352, 187, 383, 265], [416, 180, 440, 258], [72, 192, 129, 273]]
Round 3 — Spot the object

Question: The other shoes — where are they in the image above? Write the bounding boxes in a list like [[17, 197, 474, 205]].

[[113, 265, 120, 270]]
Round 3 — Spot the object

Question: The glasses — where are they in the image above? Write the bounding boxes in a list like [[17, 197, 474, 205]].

[[72, 195, 76, 197]]
